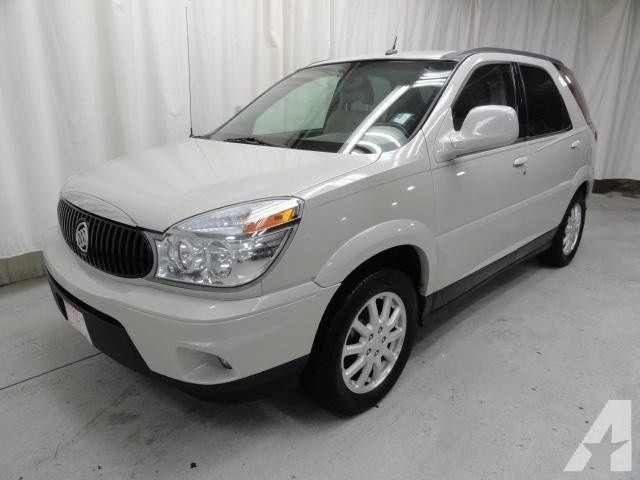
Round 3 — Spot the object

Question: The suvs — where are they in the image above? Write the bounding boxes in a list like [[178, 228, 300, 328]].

[[39, 48, 600, 423]]
